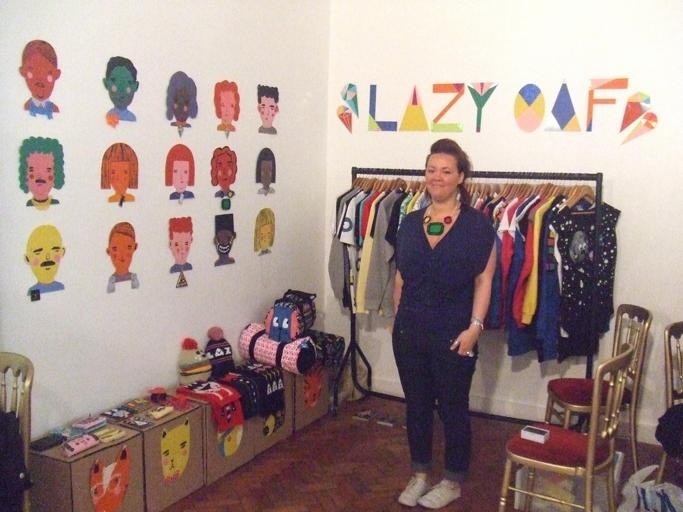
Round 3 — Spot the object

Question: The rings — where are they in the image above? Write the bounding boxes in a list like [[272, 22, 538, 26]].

[[466, 351, 474, 357]]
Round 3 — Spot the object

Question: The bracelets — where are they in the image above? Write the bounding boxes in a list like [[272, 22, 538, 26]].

[[471, 319, 483, 330]]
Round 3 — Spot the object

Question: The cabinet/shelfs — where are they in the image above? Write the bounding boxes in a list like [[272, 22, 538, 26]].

[[179, 378, 257, 488], [256, 367, 293, 456], [26, 419, 146, 512], [107, 395, 205, 512], [295, 364, 332, 432]]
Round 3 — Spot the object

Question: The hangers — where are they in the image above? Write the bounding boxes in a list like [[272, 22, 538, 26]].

[[352, 168, 606, 216]]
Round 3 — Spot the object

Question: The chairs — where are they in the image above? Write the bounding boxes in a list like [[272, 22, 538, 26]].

[[655, 321, 683, 486], [497, 343, 636, 512], [0, 352, 34, 511], [544, 303, 652, 471]]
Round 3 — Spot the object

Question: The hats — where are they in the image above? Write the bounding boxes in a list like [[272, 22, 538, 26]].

[[204, 327, 235, 379], [176, 337, 212, 388]]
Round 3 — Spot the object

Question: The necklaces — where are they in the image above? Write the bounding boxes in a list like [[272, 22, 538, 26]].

[[422, 200, 459, 237]]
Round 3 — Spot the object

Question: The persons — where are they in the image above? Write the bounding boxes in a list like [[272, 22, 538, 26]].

[[380, 137, 498, 509]]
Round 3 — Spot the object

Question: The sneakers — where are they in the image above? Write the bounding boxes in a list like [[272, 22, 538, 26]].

[[416, 481, 462, 510], [396, 473, 432, 507]]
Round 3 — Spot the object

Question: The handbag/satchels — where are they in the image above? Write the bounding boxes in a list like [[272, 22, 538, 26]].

[[237, 321, 317, 376], [306, 328, 345, 367], [264, 288, 318, 344]]
